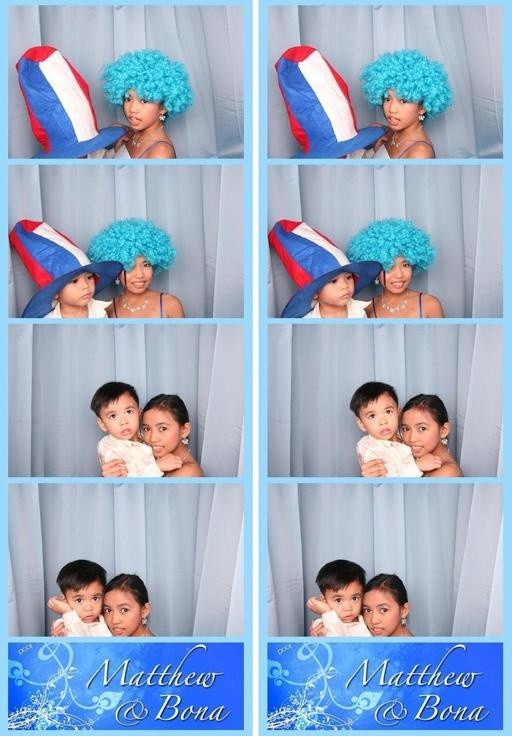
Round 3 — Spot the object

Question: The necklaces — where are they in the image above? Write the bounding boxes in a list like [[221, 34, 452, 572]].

[[390, 125, 426, 147], [131, 124, 166, 147], [380, 288, 409, 313], [120, 289, 153, 313], [441, 445, 449, 462], [181, 449, 192, 462]]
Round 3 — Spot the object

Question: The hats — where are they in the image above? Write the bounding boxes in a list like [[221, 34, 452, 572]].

[[271, 219, 385, 319], [275, 45, 386, 160], [8, 221, 125, 318], [15, 46, 128, 159]]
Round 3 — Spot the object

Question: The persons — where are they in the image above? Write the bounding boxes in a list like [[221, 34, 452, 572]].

[[363, 47, 453, 157], [357, 394, 464, 477], [90, 383, 183, 477], [309, 574, 413, 637], [50, 575, 157, 636], [349, 382, 442, 477], [77, 123, 135, 158], [302, 272, 366, 318], [100, 48, 192, 158], [89, 218, 187, 318], [44, 271, 110, 318], [99, 394, 206, 477], [47, 559, 112, 637], [306, 560, 373, 637], [340, 123, 392, 161], [346, 218, 445, 318]]
